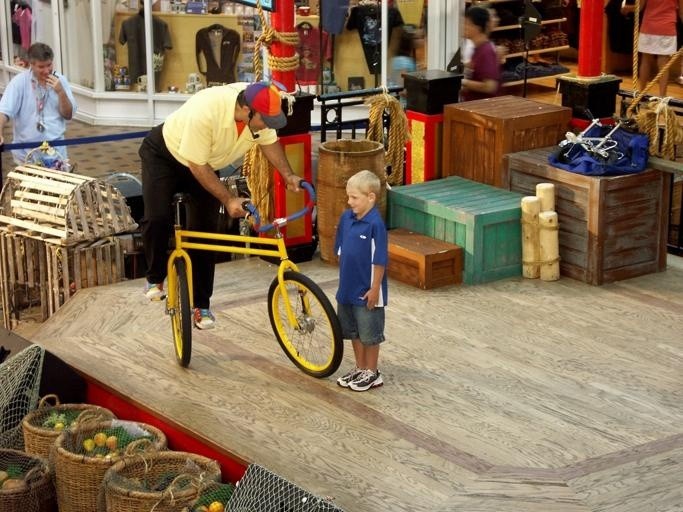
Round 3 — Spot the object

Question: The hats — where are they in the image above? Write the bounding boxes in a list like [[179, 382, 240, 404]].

[[245, 80, 287, 129]]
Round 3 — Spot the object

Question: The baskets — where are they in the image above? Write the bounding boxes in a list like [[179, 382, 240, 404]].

[[0, 449, 50, 512], [23, 395, 116, 488], [105, 440, 221, 511], [54, 419, 167, 512]]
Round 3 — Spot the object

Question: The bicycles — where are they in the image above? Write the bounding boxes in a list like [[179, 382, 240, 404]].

[[150, 179, 343, 380]]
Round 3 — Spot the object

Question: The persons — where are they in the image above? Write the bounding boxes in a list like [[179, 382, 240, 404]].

[[1, 42, 78, 173], [636, 0, 683, 98], [459, 7, 501, 100], [386, 5, 405, 63], [138, 80, 302, 330], [335, 170, 389, 392]]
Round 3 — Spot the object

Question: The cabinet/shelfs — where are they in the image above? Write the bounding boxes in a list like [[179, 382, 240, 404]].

[[467, 1, 572, 88], [94, 0, 272, 128], [0, 0, 98, 127]]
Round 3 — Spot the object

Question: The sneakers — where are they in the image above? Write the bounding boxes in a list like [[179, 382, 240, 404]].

[[193, 308, 216, 330], [337, 367, 366, 388], [143, 280, 163, 298], [348, 368, 384, 392]]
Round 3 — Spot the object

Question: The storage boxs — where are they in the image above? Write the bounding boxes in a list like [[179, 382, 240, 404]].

[[383, 227, 462, 288], [442, 96, 572, 190], [506, 144, 673, 286], [388, 177, 534, 286]]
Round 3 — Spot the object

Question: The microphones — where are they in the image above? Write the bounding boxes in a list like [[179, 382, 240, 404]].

[[248, 112, 259, 139]]
